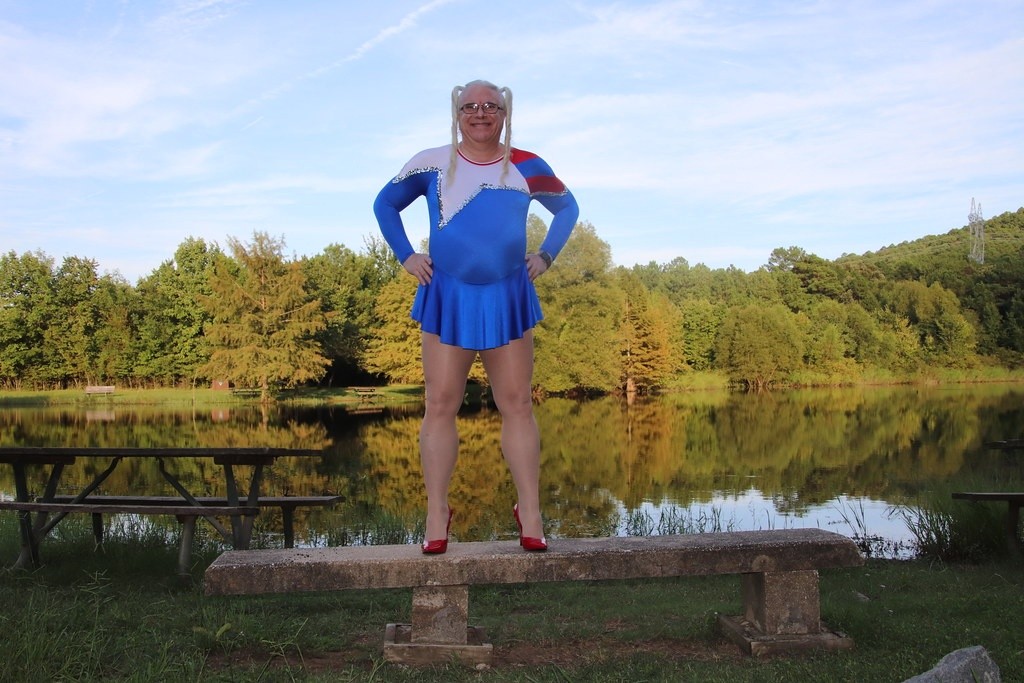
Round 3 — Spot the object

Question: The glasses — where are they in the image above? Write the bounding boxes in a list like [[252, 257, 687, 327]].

[[459, 101, 504, 114]]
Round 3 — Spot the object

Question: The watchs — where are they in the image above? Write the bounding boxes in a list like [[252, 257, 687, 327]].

[[538, 250, 552, 269]]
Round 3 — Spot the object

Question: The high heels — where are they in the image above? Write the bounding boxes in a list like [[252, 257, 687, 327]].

[[513, 503, 547, 551], [421, 504, 451, 553]]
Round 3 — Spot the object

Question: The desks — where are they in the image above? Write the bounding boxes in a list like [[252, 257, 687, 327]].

[[0, 446, 324, 573]]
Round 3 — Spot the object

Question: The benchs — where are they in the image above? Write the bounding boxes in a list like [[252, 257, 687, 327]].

[[950, 492, 1024, 554], [35, 496, 347, 561], [0, 501, 260, 579], [203, 529, 862, 667]]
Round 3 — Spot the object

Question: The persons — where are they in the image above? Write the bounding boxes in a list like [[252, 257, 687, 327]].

[[374, 79, 580, 554]]
